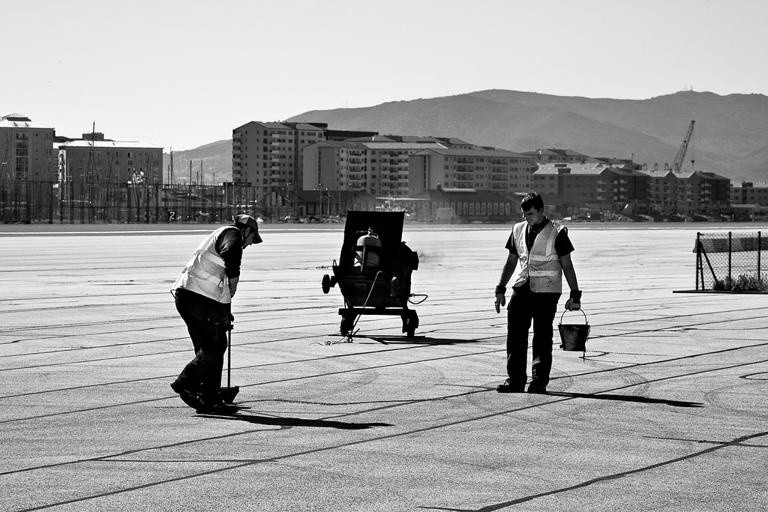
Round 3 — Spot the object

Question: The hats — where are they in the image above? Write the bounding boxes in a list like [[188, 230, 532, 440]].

[[233, 213, 263, 244]]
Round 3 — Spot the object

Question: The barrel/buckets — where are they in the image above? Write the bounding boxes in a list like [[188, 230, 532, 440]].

[[353, 226, 381, 268], [557, 308, 590, 352]]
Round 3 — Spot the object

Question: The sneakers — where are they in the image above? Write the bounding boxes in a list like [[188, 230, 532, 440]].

[[169, 379, 203, 411], [527, 380, 548, 393], [495, 379, 525, 392], [195, 396, 240, 417]]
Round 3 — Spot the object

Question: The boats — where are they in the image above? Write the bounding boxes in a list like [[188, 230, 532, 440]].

[[0, 120, 253, 224]]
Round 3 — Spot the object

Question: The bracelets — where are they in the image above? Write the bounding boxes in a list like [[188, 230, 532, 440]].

[[570, 290, 582, 304], [495, 285, 506, 294]]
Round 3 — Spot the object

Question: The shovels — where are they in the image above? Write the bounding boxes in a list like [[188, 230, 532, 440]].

[[220, 313, 239, 404], [699, 241, 725, 291]]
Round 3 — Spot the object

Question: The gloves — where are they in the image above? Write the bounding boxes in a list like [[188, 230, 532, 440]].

[[564, 289, 583, 311], [494, 285, 507, 313]]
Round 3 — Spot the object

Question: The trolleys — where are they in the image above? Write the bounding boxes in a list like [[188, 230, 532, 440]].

[[322, 203, 425, 338]]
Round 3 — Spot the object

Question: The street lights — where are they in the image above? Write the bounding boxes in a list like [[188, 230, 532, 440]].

[[286, 182, 292, 215], [314, 183, 322, 219], [325, 184, 330, 214]]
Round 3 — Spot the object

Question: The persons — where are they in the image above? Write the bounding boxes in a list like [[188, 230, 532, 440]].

[[495, 191, 582, 394], [169, 213, 265, 416]]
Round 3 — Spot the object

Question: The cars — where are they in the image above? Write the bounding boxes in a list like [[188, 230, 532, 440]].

[[254, 214, 342, 224], [559, 210, 749, 223]]
